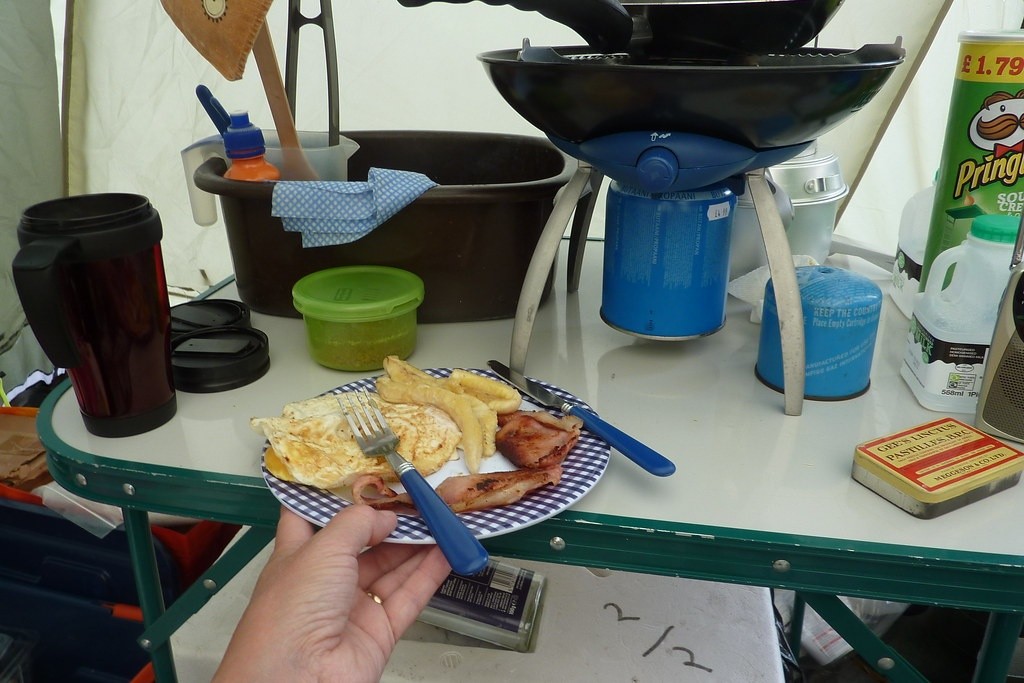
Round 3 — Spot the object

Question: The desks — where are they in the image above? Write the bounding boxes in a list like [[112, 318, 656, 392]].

[[35, 231, 1024, 683]]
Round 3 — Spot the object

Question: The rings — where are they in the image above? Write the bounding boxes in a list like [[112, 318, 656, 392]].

[[363, 589, 385, 606]]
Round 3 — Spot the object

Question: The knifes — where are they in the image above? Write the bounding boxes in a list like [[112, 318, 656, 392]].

[[487, 358, 676, 478]]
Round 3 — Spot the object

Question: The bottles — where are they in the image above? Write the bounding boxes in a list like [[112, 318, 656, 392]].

[[414, 556, 547, 652], [889, 168, 938, 319], [917, 215, 1021, 335], [221, 112, 279, 184]]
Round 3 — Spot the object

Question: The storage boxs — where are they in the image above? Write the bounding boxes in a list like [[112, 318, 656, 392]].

[[0, 369, 244, 672], [178, 127, 580, 328], [291, 264, 426, 374]]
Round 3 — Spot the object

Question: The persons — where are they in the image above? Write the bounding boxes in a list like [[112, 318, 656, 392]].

[[210, 499, 455, 683]]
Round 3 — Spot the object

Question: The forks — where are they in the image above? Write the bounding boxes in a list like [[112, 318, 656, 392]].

[[336, 385, 489, 576]]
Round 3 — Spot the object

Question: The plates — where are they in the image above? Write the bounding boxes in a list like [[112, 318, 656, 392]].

[[261, 367, 610, 546]]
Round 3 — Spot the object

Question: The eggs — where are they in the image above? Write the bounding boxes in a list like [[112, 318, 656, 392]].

[[252, 395, 461, 486]]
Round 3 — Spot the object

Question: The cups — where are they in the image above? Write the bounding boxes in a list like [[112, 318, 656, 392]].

[[11, 192, 177, 438], [179, 128, 360, 227]]
